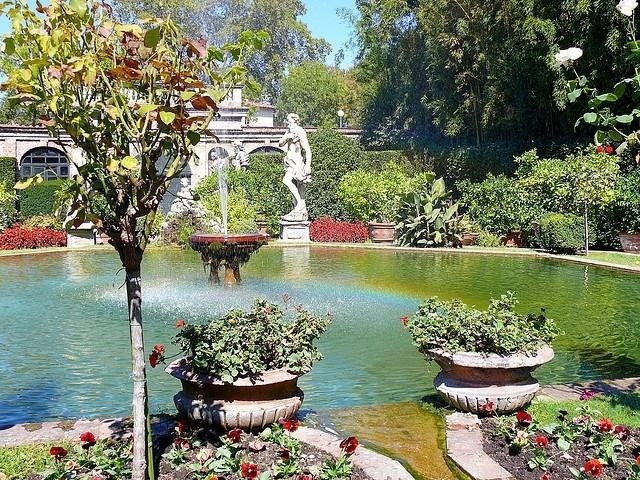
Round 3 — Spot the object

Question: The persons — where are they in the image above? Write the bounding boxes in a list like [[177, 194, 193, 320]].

[[172, 177, 195, 213], [231, 141, 248, 171], [278, 113, 312, 212], [208, 152, 222, 172]]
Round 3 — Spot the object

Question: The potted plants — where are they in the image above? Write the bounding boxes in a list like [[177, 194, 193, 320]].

[[456, 148, 640, 254], [400, 292, 564, 414], [336, 162, 432, 242]]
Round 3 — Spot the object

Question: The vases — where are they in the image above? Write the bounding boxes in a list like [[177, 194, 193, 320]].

[[164, 358, 302, 430]]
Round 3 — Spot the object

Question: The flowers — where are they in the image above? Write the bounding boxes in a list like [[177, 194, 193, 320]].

[[149, 298, 331, 384]]
